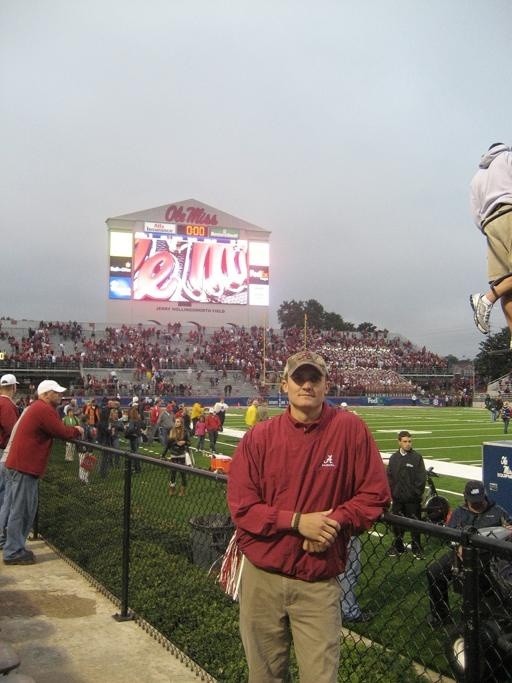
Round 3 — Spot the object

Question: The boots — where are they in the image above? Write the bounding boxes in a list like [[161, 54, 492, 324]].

[[178, 482, 186, 496], [169, 481, 177, 496]]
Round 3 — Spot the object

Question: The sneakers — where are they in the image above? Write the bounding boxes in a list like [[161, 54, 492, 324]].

[[470, 293, 493, 334], [3, 549, 34, 565], [412, 551, 426, 561], [427, 614, 451, 626], [386, 545, 405, 557]]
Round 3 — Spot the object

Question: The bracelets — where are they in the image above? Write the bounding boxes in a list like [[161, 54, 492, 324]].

[[292, 511, 300, 534]]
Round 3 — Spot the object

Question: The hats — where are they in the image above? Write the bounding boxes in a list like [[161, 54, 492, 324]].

[[38, 380, 67, 394], [288, 350, 329, 381], [63, 405, 73, 415], [463, 481, 484, 503], [0, 373, 19, 386]]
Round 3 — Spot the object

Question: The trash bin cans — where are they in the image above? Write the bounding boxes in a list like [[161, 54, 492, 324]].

[[189, 513, 234, 567]]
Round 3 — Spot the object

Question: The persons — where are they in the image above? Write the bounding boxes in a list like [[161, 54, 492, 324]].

[[226, 350, 394, 683], [1, 372, 20, 503], [1, 391, 38, 502], [1, 315, 474, 408], [468, 141, 511, 347], [484, 383, 511, 433], [59, 394, 228, 496], [338, 536, 375, 624], [425, 479, 512, 624], [242, 394, 268, 428], [385, 430, 427, 560], [1, 379, 85, 564]]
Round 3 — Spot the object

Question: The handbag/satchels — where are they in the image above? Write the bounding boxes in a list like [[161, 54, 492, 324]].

[[184, 449, 195, 467], [81, 455, 96, 471]]
